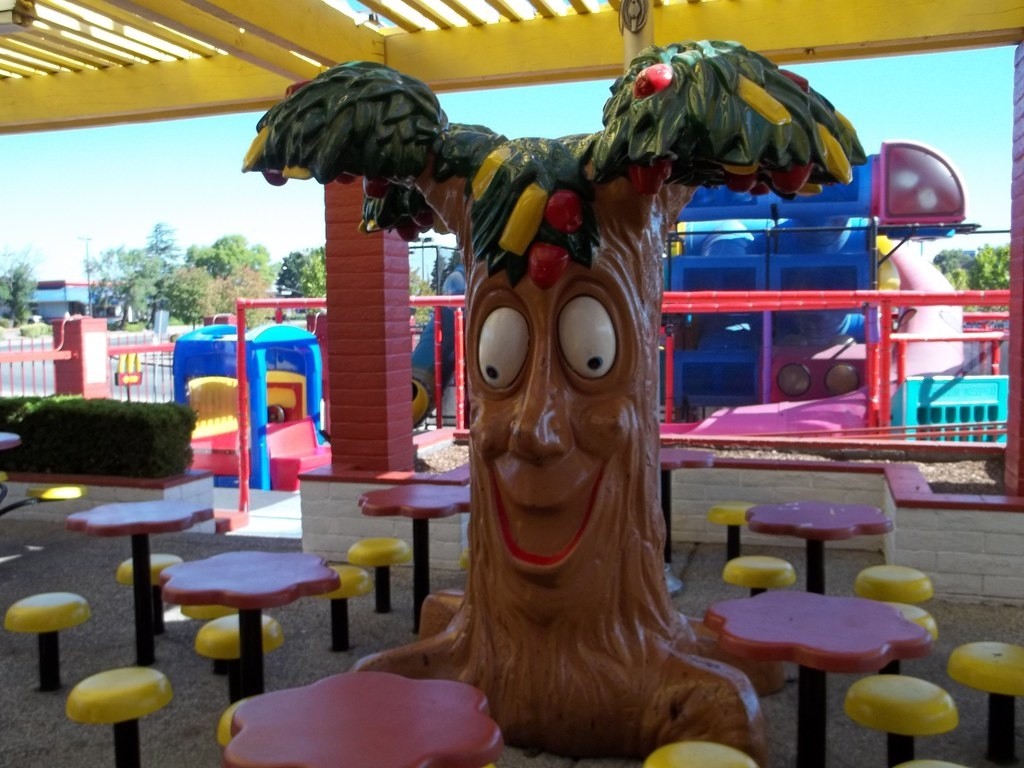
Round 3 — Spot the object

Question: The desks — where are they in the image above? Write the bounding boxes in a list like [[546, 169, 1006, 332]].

[[0, 432, 934, 768]]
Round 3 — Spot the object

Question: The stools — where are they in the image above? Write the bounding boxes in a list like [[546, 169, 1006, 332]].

[[0, 472, 1023, 768]]
